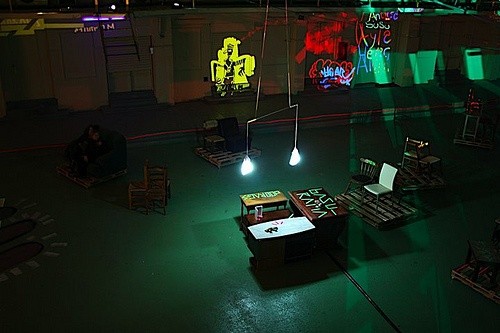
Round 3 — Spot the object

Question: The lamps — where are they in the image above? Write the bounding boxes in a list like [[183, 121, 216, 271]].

[[240, 104, 302, 176]]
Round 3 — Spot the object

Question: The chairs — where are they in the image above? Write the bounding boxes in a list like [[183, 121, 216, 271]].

[[360, 163, 399, 214], [403, 139, 444, 174], [217, 116, 252, 153], [202, 119, 224, 153], [68, 124, 128, 178], [128, 159, 171, 215], [344, 158, 379, 196]]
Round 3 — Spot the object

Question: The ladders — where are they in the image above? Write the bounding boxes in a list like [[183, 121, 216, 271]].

[[98, 9, 141, 64]]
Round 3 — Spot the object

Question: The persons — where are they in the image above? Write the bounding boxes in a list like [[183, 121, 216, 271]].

[[82, 125, 120, 174]]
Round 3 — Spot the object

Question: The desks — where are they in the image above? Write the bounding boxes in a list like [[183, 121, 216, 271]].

[[288, 187, 350, 250], [240, 191, 288, 219], [462, 112, 481, 142], [246, 216, 315, 273]]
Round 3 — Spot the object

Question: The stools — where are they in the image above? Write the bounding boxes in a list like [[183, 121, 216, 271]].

[[466, 239, 500, 282]]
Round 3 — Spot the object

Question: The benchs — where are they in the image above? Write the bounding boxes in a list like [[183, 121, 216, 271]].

[[246, 209, 292, 224]]
